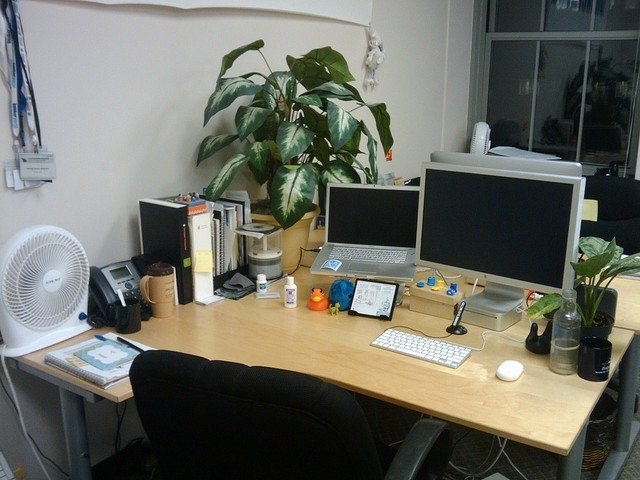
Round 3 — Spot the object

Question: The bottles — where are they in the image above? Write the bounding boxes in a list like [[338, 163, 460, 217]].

[[549, 288, 581, 375]]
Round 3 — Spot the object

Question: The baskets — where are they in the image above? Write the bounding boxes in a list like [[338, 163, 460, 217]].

[[581, 393, 619, 472]]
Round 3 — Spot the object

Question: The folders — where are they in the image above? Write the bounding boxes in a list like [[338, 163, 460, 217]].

[[139, 198, 194, 304]]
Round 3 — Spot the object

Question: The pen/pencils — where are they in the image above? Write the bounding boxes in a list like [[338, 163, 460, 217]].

[[116, 337, 145, 354], [95, 334, 123, 346]]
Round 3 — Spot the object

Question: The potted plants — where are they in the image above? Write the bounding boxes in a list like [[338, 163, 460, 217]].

[[525, 236, 640, 339], [195, 40, 394, 272]]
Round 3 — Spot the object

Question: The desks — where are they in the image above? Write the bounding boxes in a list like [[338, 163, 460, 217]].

[[1, 226, 639, 480]]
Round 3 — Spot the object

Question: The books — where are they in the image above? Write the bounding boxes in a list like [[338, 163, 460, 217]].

[[45, 332, 157, 389], [138, 197, 248, 305]]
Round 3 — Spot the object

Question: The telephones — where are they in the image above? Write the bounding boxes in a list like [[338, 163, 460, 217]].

[[87, 260, 151, 329]]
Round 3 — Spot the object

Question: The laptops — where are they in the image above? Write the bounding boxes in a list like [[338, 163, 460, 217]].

[[430, 149, 583, 184], [309, 180, 420, 283]]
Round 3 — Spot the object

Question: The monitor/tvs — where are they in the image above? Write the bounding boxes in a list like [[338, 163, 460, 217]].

[[417, 163, 588, 334]]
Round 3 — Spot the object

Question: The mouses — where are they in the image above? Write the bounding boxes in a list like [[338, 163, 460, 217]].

[[495, 357, 524, 383]]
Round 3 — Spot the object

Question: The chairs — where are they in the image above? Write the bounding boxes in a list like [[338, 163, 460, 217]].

[[129, 351, 454, 480]]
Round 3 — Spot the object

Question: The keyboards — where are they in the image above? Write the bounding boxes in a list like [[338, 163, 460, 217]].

[[369, 326, 474, 371]]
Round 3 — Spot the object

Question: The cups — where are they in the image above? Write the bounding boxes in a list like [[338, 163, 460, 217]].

[[578, 336, 612, 383], [105, 299, 141, 334], [140, 263, 175, 318]]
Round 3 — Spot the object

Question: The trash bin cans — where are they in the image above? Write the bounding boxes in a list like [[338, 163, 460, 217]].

[[558, 392, 618, 470]]
